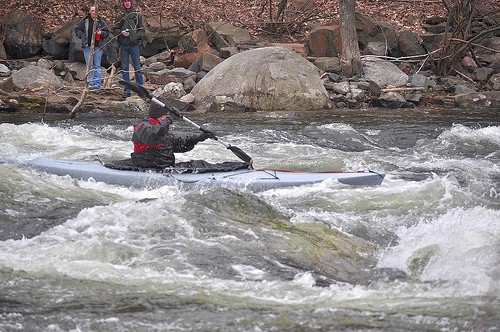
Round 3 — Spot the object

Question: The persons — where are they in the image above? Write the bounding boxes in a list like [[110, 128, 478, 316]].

[[74, 5, 109, 90], [112, 0, 146, 98], [130, 102, 250, 171]]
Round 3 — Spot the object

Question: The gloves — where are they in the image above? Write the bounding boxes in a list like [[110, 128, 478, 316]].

[[169, 107, 183, 121], [198, 129, 214, 142]]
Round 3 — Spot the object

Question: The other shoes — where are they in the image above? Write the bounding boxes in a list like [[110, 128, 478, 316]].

[[122, 93, 130, 98]]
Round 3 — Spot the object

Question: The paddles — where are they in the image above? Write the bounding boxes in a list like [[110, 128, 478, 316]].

[[118, 80, 253, 164]]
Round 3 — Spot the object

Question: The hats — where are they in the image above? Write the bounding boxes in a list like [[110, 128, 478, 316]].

[[149, 99, 171, 118]]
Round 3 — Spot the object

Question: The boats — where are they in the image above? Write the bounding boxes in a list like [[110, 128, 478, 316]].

[[0, 151, 387, 192]]
